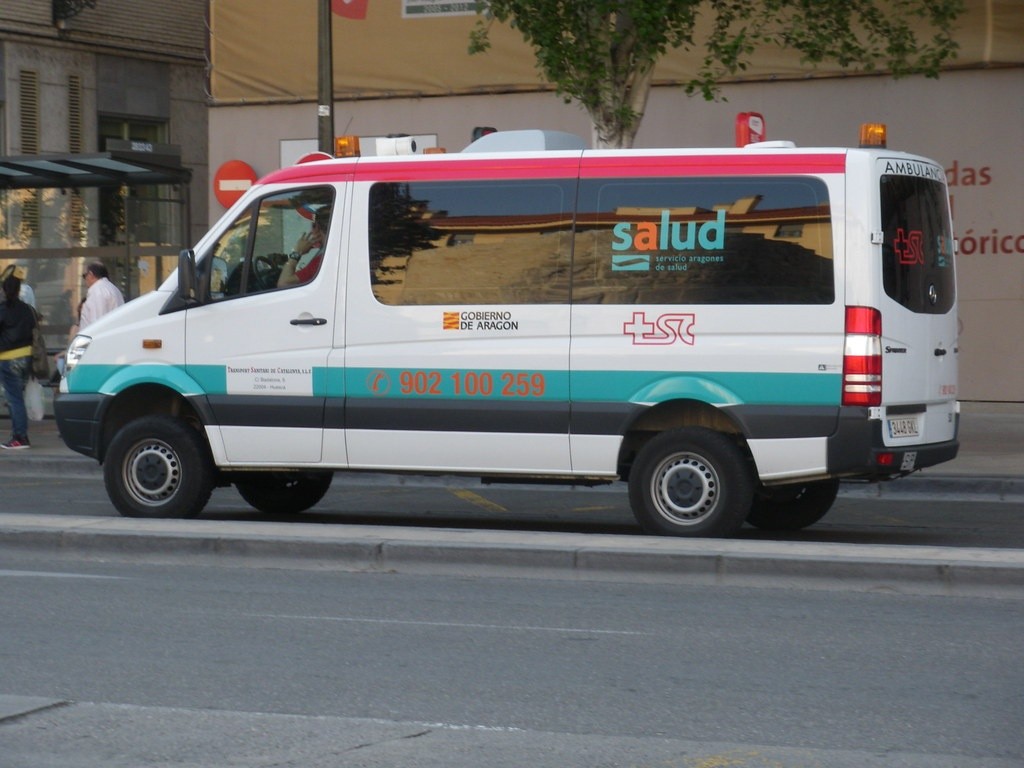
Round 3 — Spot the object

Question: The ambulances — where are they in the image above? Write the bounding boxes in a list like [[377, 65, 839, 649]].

[[54, 120, 966, 538]]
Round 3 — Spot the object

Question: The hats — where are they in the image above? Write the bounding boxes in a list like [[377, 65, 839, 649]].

[[12, 265, 26, 279]]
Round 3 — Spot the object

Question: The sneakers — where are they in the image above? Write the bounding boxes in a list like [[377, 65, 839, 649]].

[[0, 433, 31, 449]]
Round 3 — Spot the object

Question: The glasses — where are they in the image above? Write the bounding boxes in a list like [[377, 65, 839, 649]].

[[311, 222, 319, 228], [82, 273, 88, 279]]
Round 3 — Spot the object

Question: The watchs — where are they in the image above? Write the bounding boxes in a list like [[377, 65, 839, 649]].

[[289, 251, 301, 262]]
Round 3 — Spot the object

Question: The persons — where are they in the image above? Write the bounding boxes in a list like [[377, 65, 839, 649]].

[[0, 266, 44, 450], [275, 205, 332, 289], [54, 262, 126, 374]]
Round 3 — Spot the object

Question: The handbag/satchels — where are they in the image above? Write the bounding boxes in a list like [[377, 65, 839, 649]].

[[23, 376, 44, 421], [27, 304, 50, 379]]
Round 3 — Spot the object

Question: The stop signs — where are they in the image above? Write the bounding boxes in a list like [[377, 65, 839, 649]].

[[213, 160, 257, 210]]
